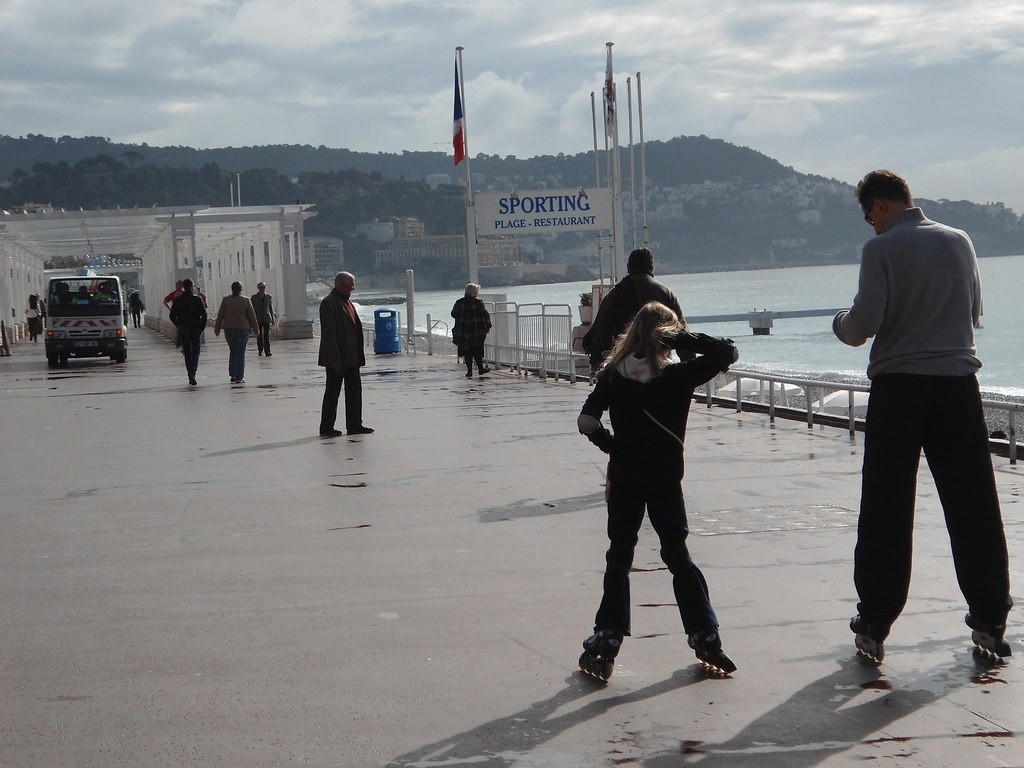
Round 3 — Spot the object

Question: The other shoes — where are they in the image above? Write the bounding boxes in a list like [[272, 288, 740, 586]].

[[319, 428, 342, 436], [258, 350, 263, 355], [478, 366, 491, 375], [347, 426, 373, 435], [266, 352, 271, 356], [465, 371, 472, 376], [230, 377, 246, 384], [189, 377, 197, 385]]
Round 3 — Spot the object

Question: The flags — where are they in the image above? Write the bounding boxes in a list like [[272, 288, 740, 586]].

[[452, 59, 465, 166], [604, 51, 615, 138]]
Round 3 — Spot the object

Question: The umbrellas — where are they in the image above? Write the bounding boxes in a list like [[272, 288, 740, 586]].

[[716, 378, 804, 397], [812, 389, 870, 415], [779, 382, 788, 407]]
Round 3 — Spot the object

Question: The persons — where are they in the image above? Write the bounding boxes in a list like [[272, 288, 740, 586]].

[[198, 287, 207, 307], [214, 281, 260, 383], [576, 300, 739, 681], [169, 278, 207, 385], [129, 293, 143, 328], [318, 272, 374, 437], [163, 280, 185, 348], [583, 248, 695, 370], [450, 284, 492, 376], [24, 294, 47, 342], [251, 282, 275, 357], [53, 283, 117, 305], [833, 170, 1013, 665]]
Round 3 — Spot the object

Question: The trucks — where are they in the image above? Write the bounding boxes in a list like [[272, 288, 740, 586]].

[[30, 276, 141, 367]]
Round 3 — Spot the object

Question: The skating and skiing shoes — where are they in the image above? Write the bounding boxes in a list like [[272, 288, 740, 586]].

[[965, 612, 1013, 666], [688, 628, 737, 678], [849, 615, 890, 664], [577, 625, 624, 681]]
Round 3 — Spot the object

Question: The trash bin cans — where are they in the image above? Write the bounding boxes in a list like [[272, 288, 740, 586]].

[[373, 307, 401, 354]]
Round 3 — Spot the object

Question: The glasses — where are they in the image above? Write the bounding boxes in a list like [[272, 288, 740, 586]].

[[865, 203, 874, 226], [258, 287, 266, 289]]
